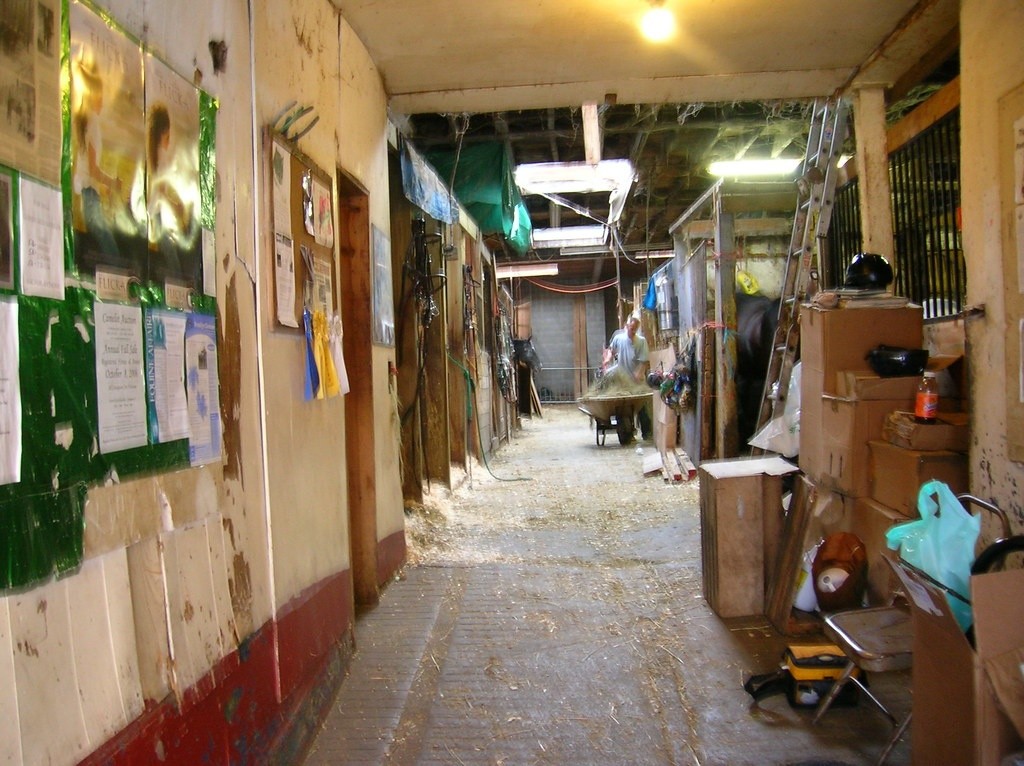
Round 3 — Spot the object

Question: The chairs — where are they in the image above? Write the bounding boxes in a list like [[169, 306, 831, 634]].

[[811, 491, 1014, 766]]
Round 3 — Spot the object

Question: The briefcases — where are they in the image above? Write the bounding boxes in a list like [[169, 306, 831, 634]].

[[746, 645, 858, 713]]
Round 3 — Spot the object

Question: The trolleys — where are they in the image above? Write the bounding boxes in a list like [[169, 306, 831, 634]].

[[576, 392, 653, 447]]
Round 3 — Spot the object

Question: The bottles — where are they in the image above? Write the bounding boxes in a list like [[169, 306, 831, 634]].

[[914, 372, 939, 425]]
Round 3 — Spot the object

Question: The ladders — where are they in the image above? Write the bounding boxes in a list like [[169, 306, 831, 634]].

[[750, 95, 851, 459]]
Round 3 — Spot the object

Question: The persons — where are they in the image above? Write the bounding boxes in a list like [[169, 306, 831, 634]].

[[602, 318, 653, 445]]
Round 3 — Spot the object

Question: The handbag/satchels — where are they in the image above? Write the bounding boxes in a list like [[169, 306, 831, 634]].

[[885, 480, 982, 636], [793, 531, 868, 613]]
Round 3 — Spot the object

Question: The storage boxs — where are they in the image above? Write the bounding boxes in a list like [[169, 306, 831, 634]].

[[699, 294, 968, 622], [879, 551, 1024, 766]]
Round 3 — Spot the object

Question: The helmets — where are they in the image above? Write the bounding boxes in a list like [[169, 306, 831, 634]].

[[844, 252, 893, 290], [864, 343, 929, 377]]
